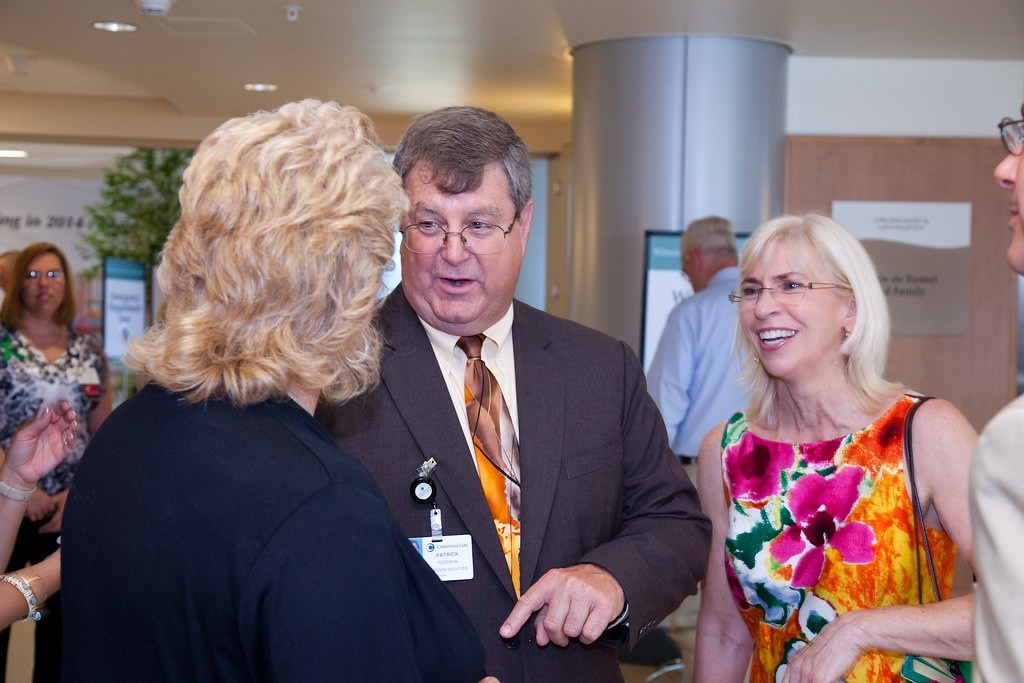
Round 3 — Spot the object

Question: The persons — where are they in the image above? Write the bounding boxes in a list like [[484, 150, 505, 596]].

[[0, 242, 112, 683], [647, 216, 751, 683], [62, 95, 502, 683], [319, 104, 712, 683], [692, 212, 978, 683], [967, 104, 1024, 683], [0, 399, 77, 630]]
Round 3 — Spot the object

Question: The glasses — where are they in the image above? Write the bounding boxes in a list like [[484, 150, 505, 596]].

[[728, 279, 852, 311], [999, 117, 1024, 156], [400, 211, 519, 255], [22, 269, 66, 286], [680, 247, 701, 279]]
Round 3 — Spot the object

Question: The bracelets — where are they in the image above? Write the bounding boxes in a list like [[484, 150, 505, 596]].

[[0, 481, 37, 501], [0, 568, 48, 622]]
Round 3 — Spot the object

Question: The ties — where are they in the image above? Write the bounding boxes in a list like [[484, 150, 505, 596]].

[[456, 333, 521, 602]]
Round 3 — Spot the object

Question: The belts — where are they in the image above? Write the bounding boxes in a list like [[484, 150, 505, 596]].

[[677, 455, 700, 467]]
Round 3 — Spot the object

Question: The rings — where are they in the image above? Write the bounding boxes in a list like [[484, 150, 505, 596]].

[[61, 431, 68, 442]]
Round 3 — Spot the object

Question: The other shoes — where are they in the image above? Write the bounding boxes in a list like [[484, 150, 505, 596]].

[[618, 626, 682, 666]]
